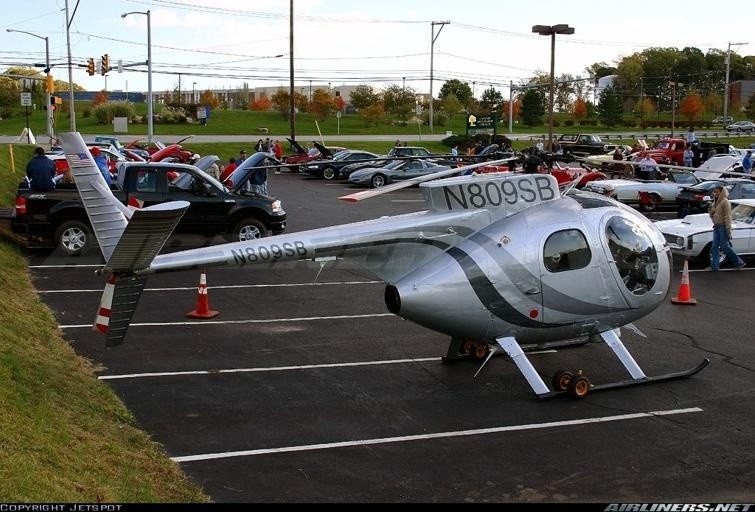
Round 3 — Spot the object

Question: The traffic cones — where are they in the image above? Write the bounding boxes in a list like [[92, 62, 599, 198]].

[[184, 268, 219, 318], [672, 261, 698, 305]]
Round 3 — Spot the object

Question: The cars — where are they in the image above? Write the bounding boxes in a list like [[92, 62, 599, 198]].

[[652, 198, 755, 268]]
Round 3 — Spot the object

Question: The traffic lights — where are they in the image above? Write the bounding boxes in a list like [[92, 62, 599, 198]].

[[44, 75, 53, 94], [101, 54, 108, 75], [87, 58, 94, 76], [52, 95, 62, 105]]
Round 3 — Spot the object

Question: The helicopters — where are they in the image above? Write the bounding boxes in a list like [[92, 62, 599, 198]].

[[56, 131, 755, 401]]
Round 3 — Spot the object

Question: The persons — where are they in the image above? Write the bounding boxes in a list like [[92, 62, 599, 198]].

[[90, 146, 113, 190], [608, 127, 698, 180], [743, 150, 754, 179], [451, 135, 563, 174], [707, 143, 718, 159], [25, 146, 58, 193], [219, 137, 283, 196], [395, 139, 402, 146], [704, 185, 747, 272]]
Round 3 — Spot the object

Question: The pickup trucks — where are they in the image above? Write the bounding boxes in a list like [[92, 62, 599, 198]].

[[0, 151, 287, 257]]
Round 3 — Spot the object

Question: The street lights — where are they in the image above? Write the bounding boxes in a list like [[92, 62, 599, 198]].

[[120, 10, 153, 143], [668, 81, 684, 139], [300, 87, 305, 112], [6, 30, 51, 136], [309, 80, 312, 112], [328, 83, 331, 112], [490, 84, 494, 112], [402, 77, 406, 116], [473, 82, 476, 98], [192, 82, 197, 105], [721, 41, 749, 121], [532, 25, 575, 153]]
